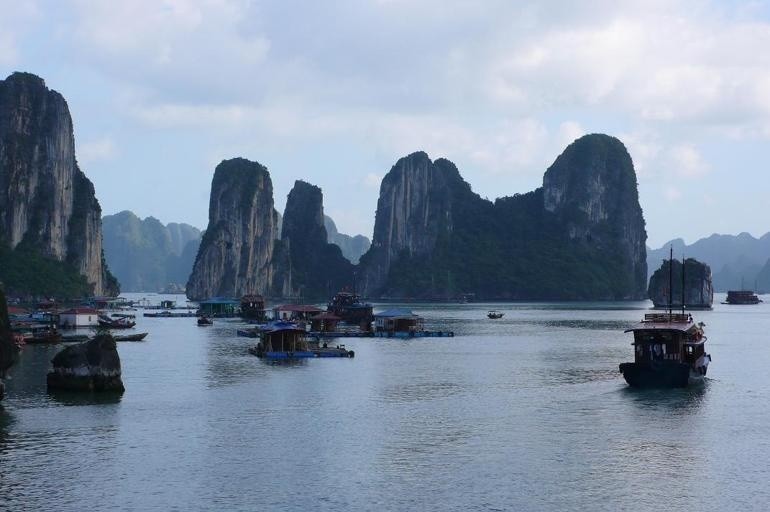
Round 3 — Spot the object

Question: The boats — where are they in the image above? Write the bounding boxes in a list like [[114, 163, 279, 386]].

[[487, 310, 504, 319], [619, 247, 711, 389], [726, 278, 763, 304], [157, 279, 186, 294]]
